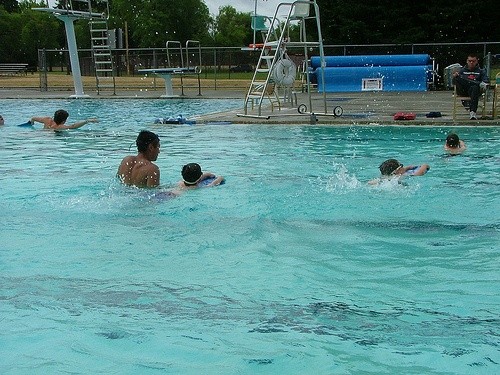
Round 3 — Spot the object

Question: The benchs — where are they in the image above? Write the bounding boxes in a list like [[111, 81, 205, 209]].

[[0, 64, 28, 77]]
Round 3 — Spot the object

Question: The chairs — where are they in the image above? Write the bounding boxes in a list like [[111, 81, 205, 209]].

[[245, 82, 280, 112], [251, 14, 268, 31], [453, 73, 500, 120], [284, 0, 310, 18]]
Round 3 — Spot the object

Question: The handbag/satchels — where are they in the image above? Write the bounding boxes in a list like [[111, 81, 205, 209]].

[[393, 111, 416, 120]]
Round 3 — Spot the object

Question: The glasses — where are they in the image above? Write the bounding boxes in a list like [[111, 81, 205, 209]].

[[395, 164, 403, 173], [201, 172, 203, 176], [156, 144, 160, 149], [467, 59, 476, 63]]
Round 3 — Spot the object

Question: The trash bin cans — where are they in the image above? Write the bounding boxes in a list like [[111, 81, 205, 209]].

[[444, 64, 463, 89]]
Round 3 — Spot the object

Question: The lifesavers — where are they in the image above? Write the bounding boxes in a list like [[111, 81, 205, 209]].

[[273, 60, 296, 87]]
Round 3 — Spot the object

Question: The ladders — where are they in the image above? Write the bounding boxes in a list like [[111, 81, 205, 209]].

[[245, 1, 327, 115], [89, 20, 115, 94]]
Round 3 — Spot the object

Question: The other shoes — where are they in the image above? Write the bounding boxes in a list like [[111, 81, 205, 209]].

[[469, 111, 478, 120], [479, 81, 489, 91]]
